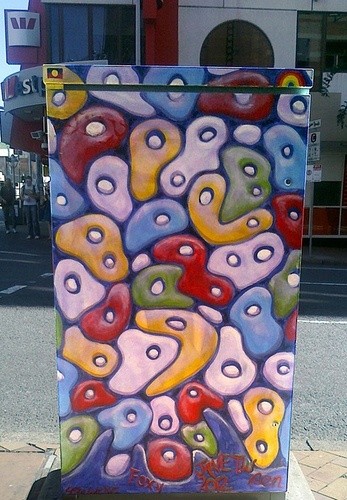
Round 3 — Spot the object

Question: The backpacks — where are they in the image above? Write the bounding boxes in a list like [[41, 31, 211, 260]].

[[23, 186, 40, 202]]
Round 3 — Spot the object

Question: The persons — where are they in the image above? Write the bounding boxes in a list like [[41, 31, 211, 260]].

[[20, 176, 40, 239], [44, 181, 51, 239], [0, 178, 17, 233]]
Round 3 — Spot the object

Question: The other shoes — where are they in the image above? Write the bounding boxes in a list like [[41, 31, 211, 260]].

[[12, 228, 16, 233], [27, 236, 31, 239], [6, 230, 11, 233], [35, 236, 40, 239]]
